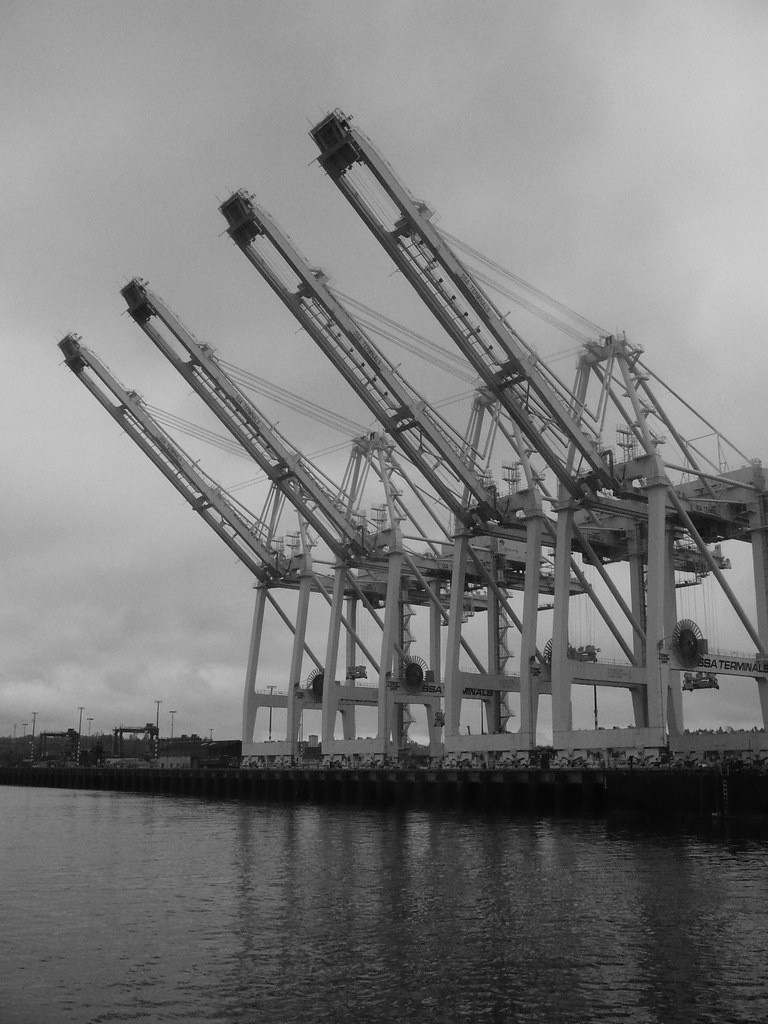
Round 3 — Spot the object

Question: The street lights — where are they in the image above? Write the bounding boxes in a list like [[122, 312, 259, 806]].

[[169, 710, 176, 740], [154, 701, 163, 760], [209, 728, 213, 737], [78, 707, 84, 763], [31, 712, 38, 760], [21, 724, 28, 741], [267, 686, 276, 742], [87, 717, 93, 741]]
